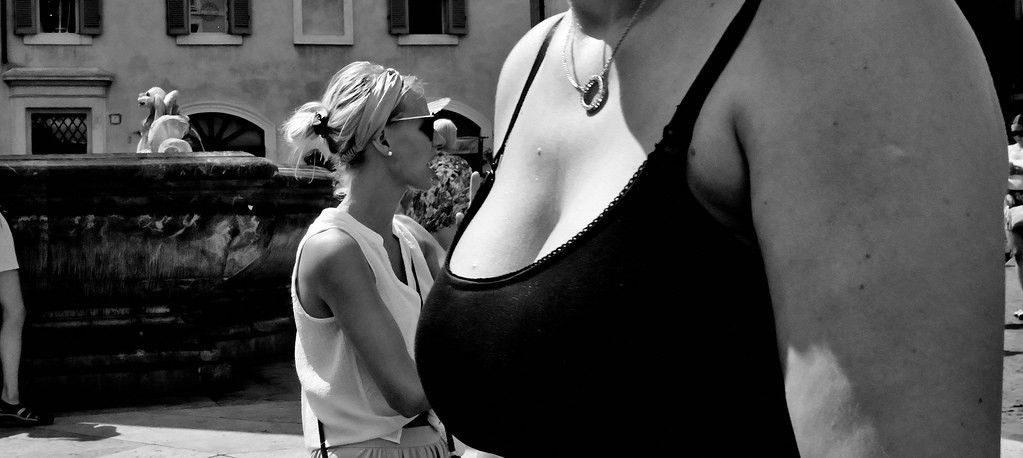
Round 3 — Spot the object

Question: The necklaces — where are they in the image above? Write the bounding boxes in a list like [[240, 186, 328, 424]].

[[557, 0, 657, 112]]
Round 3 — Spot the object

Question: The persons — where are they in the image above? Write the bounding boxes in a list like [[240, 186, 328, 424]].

[[1007, 114, 1023, 331], [278, 60, 480, 458], [395, 118, 473, 253], [414, 0, 1005, 458], [0, 211, 39, 434]]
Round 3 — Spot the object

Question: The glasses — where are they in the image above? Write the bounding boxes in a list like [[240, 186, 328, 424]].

[[388, 112, 433, 142]]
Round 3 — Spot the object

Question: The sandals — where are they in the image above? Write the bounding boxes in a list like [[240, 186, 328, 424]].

[[0, 407, 38, 422]]
